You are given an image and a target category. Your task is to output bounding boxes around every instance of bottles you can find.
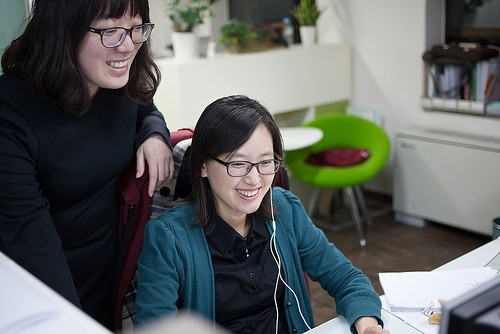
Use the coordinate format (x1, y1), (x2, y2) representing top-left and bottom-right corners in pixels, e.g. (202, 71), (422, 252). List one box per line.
(281, 17), (294, 50)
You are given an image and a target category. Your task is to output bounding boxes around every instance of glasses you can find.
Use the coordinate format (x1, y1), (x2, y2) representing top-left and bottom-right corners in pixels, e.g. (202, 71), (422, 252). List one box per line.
(88, 20), (156, 48)
(213, 152), (284, 177)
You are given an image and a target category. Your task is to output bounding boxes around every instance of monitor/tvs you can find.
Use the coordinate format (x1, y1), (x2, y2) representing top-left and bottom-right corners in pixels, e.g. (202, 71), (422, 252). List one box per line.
(435, 276), (500, 334)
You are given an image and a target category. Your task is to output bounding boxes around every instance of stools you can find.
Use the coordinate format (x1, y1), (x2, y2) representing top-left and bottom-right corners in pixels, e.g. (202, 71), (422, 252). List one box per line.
(284, 112), (390, 247)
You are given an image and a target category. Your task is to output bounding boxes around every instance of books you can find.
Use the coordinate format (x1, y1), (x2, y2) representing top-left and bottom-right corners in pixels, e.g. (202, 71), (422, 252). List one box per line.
(427, 58), (500, 103)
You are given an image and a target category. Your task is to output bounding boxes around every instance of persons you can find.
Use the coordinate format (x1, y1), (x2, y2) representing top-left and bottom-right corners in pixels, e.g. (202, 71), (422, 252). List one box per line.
(131, 95), (392, 334)
(0, 0), (176, 331)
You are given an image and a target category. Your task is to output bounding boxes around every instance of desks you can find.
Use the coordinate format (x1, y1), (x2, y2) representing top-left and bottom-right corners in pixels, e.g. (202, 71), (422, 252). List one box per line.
(303, 234), (500, 334)
(173, 124), (323, 155)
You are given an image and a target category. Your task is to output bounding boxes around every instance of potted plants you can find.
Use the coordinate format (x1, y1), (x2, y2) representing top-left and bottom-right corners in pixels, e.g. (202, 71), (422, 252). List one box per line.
(164, 0), (219, 60)
(290, 0), (323, 46)
(218, 17), (249, 54)
